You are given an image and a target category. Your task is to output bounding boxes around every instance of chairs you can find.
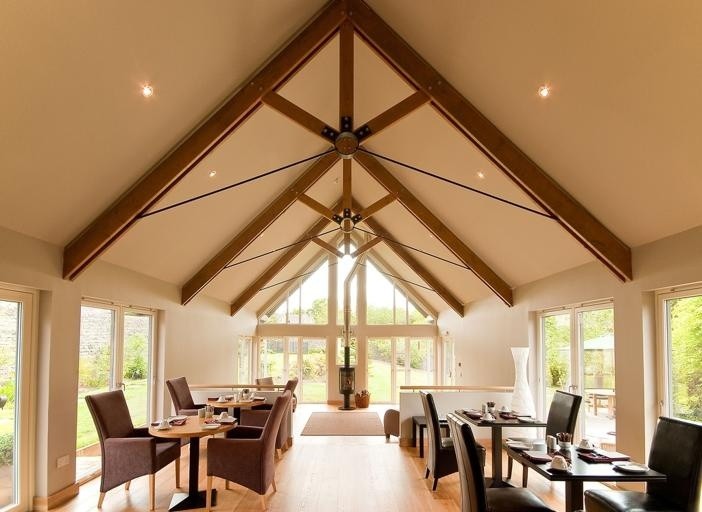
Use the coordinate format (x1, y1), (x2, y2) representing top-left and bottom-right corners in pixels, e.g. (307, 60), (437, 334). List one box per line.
(75, 366), (301, 512)
(584, 417), (702, 512)
(446, 412), (554, 512)
(421, 390), (485, 490)
(506, 390), (582, 488)
(384, 409), (399, 441)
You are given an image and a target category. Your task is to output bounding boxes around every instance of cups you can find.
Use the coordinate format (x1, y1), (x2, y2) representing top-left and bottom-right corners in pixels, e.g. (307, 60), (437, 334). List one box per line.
(217, 392), (265, 403)
(484, 413), (493, 418)
(546, 436), (556, 454)
(481, 403), (486, 412)
(156, 415), (169, 427)
(580, 438), (593, 447)
(552, 456), (566, 468)
(216, 407), (232, 418)
(196, 404), (214, 421)
(502, 405), (509, 410)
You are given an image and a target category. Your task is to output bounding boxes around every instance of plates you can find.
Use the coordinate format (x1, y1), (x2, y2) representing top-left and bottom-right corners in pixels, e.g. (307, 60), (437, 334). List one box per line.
(211, 418), (238, 424)
(168, 414), (189, 423)
(505, 434), (596, 471)
(461, 403), (542, 423)
(609, 460), (649, 473)
(154, 427), (173, 431)
(237, 400), (254, 402)
(254, 396), (264, 400)
(201, 423), (224, 429)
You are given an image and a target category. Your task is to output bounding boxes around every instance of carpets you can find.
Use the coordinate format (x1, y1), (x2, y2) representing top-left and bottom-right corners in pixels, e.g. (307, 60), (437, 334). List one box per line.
(301, 403), (388, 439)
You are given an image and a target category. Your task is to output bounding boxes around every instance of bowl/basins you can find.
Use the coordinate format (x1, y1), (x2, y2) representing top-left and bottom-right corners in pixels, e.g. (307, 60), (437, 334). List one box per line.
(488, 406), (495, 412)
(558, 441), (572, 450)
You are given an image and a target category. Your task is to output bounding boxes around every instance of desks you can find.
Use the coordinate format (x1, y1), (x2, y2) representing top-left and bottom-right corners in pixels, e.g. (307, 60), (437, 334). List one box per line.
(412, 416), (448, 459)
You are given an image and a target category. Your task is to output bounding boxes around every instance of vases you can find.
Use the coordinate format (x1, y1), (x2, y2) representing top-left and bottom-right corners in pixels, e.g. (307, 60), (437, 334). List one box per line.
(511, 345), (536, 444)
